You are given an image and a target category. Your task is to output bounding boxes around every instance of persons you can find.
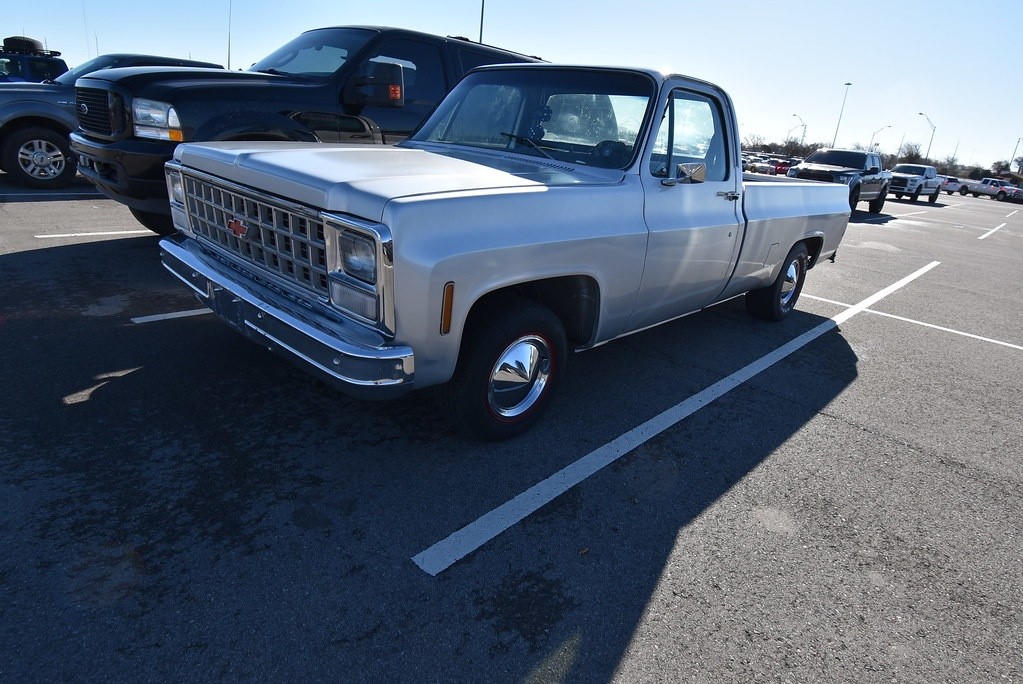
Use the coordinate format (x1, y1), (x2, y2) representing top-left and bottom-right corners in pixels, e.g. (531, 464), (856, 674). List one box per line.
(5, 62), (18, 76)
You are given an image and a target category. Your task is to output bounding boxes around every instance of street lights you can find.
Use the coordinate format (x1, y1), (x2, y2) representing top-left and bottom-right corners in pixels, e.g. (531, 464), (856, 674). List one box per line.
(1006, 137), (1023, 172)
(866, 124), (892, 153)
(918, 113), (937, 166)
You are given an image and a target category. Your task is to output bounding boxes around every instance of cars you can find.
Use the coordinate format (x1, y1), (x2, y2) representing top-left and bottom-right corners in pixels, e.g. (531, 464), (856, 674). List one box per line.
(788, 158), (802, 167)
(766, 158), (791, 177)
(742, 156), (776, 176)
(740, 151), (790, 164)
(618, 138), (713, 156)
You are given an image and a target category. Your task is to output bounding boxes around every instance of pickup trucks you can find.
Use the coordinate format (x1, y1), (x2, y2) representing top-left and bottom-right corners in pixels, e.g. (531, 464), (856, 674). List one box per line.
(157, 64), (852, 442)
(786, 149), (893, 215)
(960, 178), (1023, 203)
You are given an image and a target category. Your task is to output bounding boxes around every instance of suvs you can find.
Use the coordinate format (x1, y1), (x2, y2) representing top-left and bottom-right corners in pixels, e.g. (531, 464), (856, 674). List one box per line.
(70, 0), (620, 237)
(888, 164), (941, 204)
(935, 175), (961, 195)
(0, 54), (225, 191)
(0, 36), (70, 83)
